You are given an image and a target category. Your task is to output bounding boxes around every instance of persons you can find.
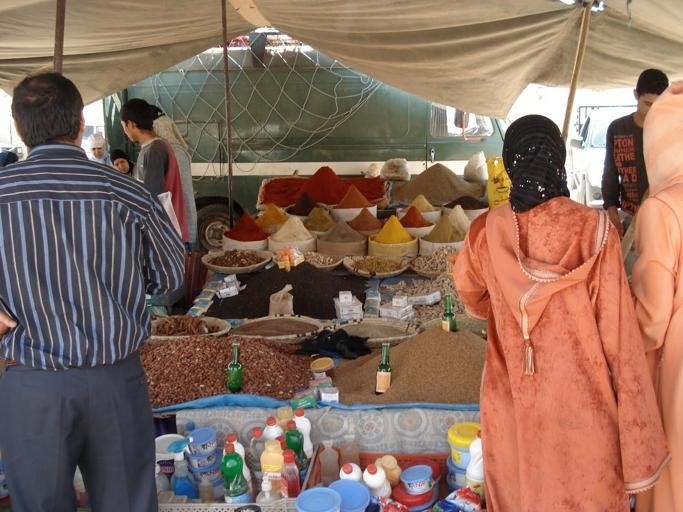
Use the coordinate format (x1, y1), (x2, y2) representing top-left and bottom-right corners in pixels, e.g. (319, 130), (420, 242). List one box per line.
(120, 96), (188, 245)
(602, 69), (683, 242)
(85, 132), (111, 165)
(150, 105), (197, 314)
(0, 71), (188, 510)
(453, 115), (671, 511)
(109, 148), (137, 177)
(626, 81), (681, 510)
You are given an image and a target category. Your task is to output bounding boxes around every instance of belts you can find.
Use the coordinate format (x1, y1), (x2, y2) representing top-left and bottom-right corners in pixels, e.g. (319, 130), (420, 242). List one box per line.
(7, 357), (18, 367)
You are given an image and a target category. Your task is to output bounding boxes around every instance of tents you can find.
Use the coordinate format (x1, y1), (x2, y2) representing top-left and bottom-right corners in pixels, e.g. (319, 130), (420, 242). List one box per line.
(0, 1), (683, 118)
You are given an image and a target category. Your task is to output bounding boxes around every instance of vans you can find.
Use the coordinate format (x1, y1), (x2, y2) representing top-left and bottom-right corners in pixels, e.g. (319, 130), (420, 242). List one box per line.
(79, 113), (105, 163)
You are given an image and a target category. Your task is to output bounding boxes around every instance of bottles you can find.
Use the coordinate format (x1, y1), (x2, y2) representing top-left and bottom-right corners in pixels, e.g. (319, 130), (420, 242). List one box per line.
(255, 477), (281, 503)
(375, 342), (391, 395)
(154, 464), (169, 495)
(262, 417), (283, 440)
(285, 421), (307, 464)
(275, 434), (287, 451)
(276, 406), (291, 430)
(259, 440), (283, 480)
(339, 463), (361, 483)
(375, 455), (401, 489)
(339, 434), (361, 464)
(291, 410), (313, 459)
(220, 444), (250, 503)
(227, 341), (242, 395)
(319, 440), (338, 486)
(222, 433), (253, 494)
(199, 473), (211, 501)
(249, 428), (264, 460)
(362, 464), (391, 500)
(169, 450), (199, 499)
(464, 430), (485, 503)
(281, 449), (300, 497)
(253, 466), (261, 495)
(442, 293), (455, 332)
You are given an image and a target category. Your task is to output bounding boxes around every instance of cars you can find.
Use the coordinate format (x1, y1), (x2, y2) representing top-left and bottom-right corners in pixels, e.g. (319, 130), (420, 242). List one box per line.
(565, 103), (636, 208)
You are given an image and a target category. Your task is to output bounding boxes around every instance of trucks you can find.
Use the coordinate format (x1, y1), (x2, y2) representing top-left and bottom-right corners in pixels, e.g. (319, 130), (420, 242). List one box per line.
(104, 30), (510, 252)
